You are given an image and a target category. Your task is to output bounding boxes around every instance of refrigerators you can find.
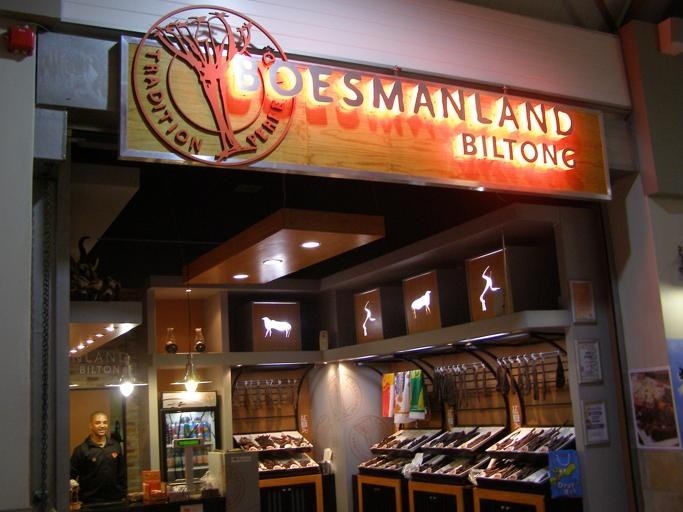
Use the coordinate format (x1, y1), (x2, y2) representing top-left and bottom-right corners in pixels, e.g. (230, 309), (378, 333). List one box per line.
(159, 390), (219, 481)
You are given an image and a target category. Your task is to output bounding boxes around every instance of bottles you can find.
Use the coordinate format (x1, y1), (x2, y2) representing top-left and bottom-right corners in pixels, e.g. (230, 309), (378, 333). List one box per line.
(166, 417), (212, 481)
(193, 327), (207, 352)
(165, 327), (177, 352)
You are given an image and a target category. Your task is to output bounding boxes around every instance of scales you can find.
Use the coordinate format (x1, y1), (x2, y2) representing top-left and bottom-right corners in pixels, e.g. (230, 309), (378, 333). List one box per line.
(166, 437), (208, 493)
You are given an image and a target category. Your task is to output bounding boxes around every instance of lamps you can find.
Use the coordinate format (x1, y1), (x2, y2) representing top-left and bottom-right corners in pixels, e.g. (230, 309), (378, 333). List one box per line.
(104, 335), (147, 398)
(170, 251), (210, 392)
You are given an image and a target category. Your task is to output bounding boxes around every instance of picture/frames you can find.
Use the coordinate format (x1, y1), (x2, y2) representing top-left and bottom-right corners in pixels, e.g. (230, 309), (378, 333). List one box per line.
(568, 279), (612, 450)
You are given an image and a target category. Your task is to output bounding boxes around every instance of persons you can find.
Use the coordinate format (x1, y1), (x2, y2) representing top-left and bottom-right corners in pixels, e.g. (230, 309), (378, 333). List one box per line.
(70, 411), (127, 504)
